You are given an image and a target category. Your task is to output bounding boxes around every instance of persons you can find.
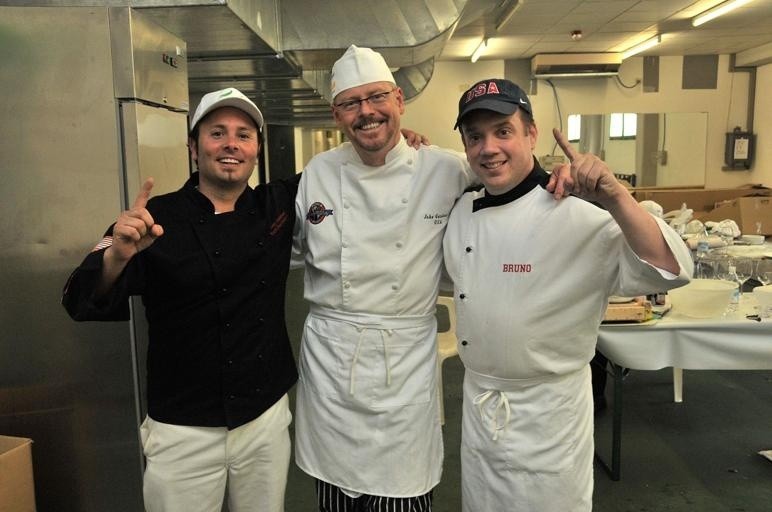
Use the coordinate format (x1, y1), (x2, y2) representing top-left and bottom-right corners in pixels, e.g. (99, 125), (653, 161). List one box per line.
(442, 77), (694, 511)
(289, 43), (589, 510)
(62, 86), (430, 512)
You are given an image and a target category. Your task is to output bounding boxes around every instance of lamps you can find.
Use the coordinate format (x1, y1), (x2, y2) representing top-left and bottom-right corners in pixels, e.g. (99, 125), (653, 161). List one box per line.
(691, 0), (754, 27)
(496, 0), (523, 31)
(622, 34), (661, 60)
(471, 39), (488, 64)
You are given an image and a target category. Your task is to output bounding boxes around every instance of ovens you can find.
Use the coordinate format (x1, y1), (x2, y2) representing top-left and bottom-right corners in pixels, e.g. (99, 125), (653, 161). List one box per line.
(0, 4), (187, 512)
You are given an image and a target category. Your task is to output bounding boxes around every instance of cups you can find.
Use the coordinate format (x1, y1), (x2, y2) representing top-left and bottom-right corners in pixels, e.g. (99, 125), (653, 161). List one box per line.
(753, 284), (772, 309)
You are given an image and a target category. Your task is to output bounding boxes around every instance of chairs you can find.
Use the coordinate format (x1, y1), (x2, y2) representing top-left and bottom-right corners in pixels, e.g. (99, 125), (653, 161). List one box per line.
(422, 295), (459, 427)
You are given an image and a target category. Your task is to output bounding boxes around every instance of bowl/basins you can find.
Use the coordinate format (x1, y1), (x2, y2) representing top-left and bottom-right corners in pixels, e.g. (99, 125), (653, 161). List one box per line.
(742, 234), (766, 245)
(671, 278), (740, 318)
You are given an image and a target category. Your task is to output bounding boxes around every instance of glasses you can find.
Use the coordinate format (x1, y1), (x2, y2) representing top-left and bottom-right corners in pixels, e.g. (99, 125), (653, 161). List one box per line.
(334, 90), (398, 109)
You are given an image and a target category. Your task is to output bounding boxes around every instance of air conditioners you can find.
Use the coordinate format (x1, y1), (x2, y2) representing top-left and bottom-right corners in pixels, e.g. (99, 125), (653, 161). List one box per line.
(530, 52), (622, 79)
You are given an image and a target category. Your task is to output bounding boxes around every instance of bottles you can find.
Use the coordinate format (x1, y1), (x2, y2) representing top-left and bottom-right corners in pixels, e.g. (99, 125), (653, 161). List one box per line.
(724, 266), (741, 316)
(697, 225), (708, 258)
(647, 292), (665, 305)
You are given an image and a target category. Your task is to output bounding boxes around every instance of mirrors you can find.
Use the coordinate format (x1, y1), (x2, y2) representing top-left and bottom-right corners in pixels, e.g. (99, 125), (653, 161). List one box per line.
(567, 112), (708, 188)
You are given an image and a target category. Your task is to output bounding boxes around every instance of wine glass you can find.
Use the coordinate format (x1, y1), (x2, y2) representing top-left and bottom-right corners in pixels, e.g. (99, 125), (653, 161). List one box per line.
(730, 258), (752, 306)
(756, 260), (772, 286)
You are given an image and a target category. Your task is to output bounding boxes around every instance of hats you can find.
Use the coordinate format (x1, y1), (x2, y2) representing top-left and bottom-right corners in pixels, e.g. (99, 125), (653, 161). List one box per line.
(454, 78), (534, 131)
(189, 87), (264, 135)
(330, 42), (397, 103)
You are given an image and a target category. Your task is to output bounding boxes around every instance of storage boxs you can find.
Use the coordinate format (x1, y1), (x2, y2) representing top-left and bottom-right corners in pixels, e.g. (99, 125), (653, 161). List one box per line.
(0, 435), (37, 512)
(715, 192), (772, 236)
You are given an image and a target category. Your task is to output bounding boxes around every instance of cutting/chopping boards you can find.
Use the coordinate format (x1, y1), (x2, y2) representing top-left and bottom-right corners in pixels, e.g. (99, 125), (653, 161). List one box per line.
(604, 293), (654, 325)
